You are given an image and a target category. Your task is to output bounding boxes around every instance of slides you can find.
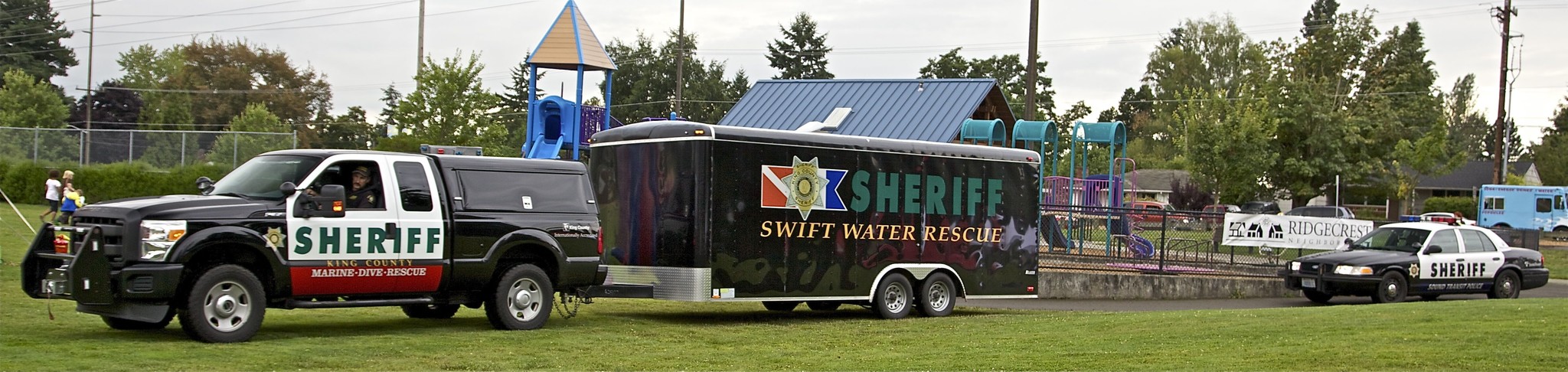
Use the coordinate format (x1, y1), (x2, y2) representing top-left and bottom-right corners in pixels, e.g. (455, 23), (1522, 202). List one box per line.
(527, 135), (564, 159)
(1040, 214), (1076, 249)
(1105, 215), (1155, 257)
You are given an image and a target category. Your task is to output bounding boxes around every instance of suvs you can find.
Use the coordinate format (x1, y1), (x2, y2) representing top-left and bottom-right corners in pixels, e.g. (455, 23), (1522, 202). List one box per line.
(1124, 201), (1195, 231)
(1201, 202), (1355, 231)
(20, 144), (608, 344)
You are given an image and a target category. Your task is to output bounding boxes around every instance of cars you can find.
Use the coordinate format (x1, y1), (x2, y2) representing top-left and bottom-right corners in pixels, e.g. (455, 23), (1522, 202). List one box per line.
(1277, 215), (1549, 303)
(1420, 213), (1476, 226)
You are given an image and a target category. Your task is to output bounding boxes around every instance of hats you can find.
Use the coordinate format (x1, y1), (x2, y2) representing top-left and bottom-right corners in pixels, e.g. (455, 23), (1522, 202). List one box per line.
(352, 167), (371, 177)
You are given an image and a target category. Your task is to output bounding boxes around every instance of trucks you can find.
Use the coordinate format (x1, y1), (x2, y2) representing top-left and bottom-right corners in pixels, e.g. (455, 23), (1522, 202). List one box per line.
(1476, 184), (1568, 241)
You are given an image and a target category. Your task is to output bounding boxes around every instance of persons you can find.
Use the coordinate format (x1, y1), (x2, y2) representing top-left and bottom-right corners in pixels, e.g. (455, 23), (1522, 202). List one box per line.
(39, 169), (88, 227)
(302, 166), (376, 210)
